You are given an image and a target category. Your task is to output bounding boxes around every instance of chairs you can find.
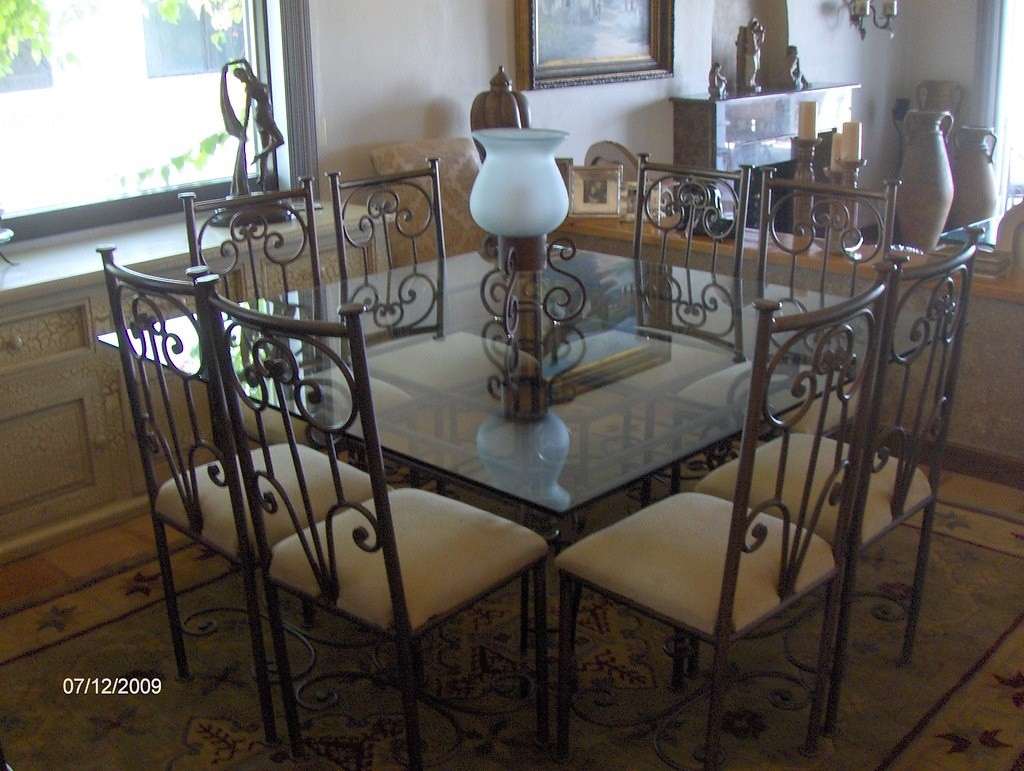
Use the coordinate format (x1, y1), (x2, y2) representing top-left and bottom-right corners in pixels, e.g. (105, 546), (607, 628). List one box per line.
(94, 152), (984, 771)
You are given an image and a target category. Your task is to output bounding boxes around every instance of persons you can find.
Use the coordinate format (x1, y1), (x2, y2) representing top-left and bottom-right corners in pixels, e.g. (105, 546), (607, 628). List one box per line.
(744, 17), (766, 86)
(228, 60), (285, 194)
(587, 180), (606, 204)
(778, 46), (812, 91)
(709, 63), (728, 100)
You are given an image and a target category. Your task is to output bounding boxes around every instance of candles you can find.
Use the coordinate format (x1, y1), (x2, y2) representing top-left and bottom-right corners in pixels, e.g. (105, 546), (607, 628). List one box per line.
(841, 122), (862, 160)
(830, 132), (850, 174)
(797, 100), (817, 140)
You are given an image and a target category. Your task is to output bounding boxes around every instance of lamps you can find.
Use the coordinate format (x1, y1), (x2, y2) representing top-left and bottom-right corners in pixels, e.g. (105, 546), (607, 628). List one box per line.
(477, 317), (585, 512)
(850, 0), (899, 40)
(470, 122), (586, 338)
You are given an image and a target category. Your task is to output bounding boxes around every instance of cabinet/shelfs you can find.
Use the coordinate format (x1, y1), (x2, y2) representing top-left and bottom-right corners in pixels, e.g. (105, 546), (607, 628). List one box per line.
(0, 198), (408, 569)
(669, 82), (863, 237)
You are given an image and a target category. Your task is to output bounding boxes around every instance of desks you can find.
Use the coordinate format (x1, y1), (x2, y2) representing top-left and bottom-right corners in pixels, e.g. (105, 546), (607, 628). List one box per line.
(95, 242), (973, 546)
(547, 211), (948, 444)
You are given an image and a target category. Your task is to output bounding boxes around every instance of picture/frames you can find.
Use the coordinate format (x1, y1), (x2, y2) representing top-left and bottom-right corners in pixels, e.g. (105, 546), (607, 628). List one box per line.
(555, 158), (572, 191)
(568, 165), (623, 218)
(618, 179), (661, 223)
(512, 0), (675, 92)
